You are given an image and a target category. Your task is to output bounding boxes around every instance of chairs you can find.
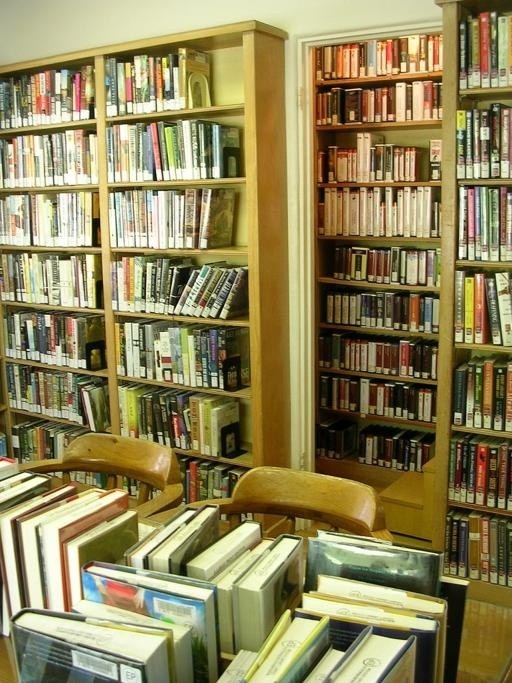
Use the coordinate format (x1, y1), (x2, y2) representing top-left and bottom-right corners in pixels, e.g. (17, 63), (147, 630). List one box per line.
(147, 464), (392, 553)
(16, 434), (184, 515)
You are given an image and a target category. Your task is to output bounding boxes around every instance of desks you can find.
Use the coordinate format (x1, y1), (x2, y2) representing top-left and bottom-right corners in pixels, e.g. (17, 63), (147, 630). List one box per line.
(1, 457), (512, 683)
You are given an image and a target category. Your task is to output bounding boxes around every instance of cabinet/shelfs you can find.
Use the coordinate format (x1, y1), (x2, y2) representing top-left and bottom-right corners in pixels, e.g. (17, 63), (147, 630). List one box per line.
(439, 1), (512, 587)
(102, 21), (294, 538)
(304, 24), (444, 489)
(1, 47), (113, 481)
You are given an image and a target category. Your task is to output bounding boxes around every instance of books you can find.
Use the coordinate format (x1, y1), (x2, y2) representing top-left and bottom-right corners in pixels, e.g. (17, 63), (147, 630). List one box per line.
(1, 456), (469, 683)
(316, 32), (444, 472)
(445, 13), (512, 587)
(104, 46), (247, 503)
(0, 66), (110, 488)
